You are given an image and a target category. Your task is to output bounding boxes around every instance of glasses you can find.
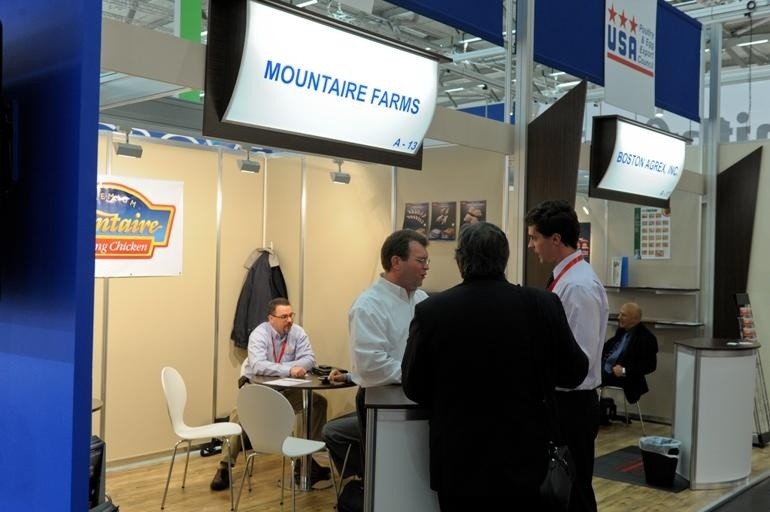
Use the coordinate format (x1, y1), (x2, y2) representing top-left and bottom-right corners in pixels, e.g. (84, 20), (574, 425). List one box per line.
(271, 312), (296, 320)
(404, 253), (432, 265)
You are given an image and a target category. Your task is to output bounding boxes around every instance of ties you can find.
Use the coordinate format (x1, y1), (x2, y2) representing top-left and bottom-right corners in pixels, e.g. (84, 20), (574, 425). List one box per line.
(605, 331), (628, 374)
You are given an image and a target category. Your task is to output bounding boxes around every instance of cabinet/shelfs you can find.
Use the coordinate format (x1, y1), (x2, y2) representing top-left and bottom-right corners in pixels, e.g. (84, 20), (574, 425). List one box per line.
(600, 285), (704, 327)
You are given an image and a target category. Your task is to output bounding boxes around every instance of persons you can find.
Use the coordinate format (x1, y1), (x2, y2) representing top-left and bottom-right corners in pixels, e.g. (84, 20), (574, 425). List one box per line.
(599, 300), (659, 386)
(209, 298), (331, 491)
(320, 367), (367, 511)
(517, 199), (611, 511)
(345, 228), (430, 441)
(400, 220), (591, 512)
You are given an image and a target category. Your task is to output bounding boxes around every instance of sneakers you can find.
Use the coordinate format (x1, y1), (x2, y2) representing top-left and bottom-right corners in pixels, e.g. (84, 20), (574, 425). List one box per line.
(209, 469), (231, 491)
(293, 457), (331, 480)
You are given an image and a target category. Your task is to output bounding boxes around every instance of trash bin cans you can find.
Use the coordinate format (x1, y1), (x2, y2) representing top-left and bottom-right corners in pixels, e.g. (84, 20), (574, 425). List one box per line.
(640, 436), (682, 487)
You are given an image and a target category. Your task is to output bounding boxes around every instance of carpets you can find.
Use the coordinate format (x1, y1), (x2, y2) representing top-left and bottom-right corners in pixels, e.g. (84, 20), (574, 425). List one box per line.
(593, 445), (690, 493)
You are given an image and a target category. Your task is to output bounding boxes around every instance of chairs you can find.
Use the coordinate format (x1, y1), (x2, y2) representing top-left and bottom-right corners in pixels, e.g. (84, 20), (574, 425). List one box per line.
(235, 384), (337, 511)
(598, 387), (647, 436)
(161, 366), (252, 510)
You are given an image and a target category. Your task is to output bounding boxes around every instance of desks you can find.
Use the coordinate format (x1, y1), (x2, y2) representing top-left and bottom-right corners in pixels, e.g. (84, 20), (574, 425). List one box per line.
(250, 375), (357, 492)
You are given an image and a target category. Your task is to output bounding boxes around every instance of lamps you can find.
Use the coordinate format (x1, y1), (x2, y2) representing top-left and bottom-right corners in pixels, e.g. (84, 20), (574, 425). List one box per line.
(237, 144), (260, 173)
(329, 158), (350, 184)
(112, 124), (143, 159)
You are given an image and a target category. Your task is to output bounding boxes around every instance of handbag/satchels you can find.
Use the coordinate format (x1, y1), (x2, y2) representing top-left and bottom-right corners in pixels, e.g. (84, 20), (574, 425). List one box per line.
(541, 431), (581, 509)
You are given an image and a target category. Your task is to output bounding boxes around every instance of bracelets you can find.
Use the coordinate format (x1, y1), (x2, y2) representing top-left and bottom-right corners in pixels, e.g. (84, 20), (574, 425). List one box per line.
(621, 367), (626, 376)
